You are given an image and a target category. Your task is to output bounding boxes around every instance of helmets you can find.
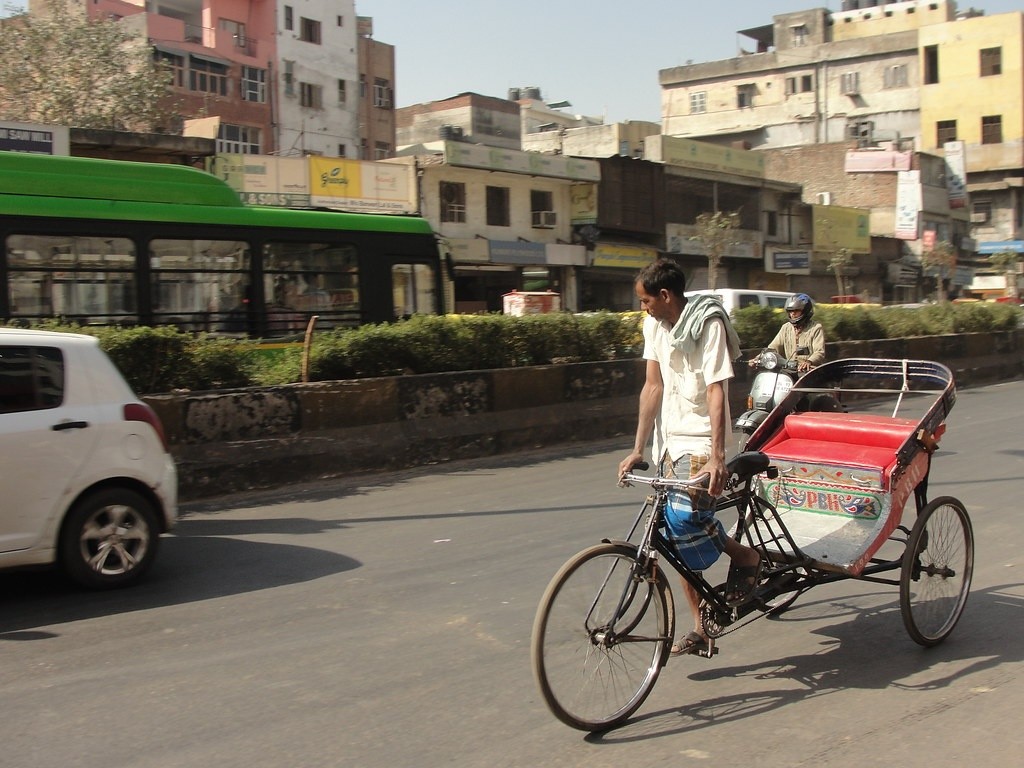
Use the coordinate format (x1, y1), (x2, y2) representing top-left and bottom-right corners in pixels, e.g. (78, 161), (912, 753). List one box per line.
(784, 293), (814, 327)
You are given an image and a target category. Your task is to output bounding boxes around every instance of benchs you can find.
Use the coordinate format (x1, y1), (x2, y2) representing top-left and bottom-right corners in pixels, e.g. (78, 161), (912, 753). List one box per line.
(756, 411), (931, 514)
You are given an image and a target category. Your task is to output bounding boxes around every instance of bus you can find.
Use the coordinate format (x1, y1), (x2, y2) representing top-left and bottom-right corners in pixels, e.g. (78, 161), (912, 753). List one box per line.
(0, 149), (460, 346)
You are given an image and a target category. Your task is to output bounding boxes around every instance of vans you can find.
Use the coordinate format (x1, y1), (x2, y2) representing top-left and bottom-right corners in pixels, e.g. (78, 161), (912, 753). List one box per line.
(684, 288), (816, 326)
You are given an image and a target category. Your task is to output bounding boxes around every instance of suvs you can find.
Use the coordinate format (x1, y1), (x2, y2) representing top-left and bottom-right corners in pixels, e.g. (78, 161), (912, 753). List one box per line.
(0, 325), (180, 594)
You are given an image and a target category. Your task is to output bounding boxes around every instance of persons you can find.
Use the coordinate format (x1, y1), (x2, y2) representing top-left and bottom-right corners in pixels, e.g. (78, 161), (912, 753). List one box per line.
(618, 259), (764, 658)
(749, 293), (825, 400)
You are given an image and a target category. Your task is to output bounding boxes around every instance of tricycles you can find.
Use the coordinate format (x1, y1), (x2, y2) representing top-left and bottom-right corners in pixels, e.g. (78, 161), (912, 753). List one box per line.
(530, 356), (976, 734)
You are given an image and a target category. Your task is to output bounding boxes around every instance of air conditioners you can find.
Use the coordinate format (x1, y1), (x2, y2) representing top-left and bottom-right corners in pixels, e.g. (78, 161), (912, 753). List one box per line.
(815, 191), (831, 206)
(531, 211), (556, 228)
(970, 213), (987, 223)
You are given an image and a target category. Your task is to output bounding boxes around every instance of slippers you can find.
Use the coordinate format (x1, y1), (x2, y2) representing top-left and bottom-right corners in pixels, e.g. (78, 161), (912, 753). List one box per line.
(725, 554), (763, 606)
(669, 631), (718, 657)
